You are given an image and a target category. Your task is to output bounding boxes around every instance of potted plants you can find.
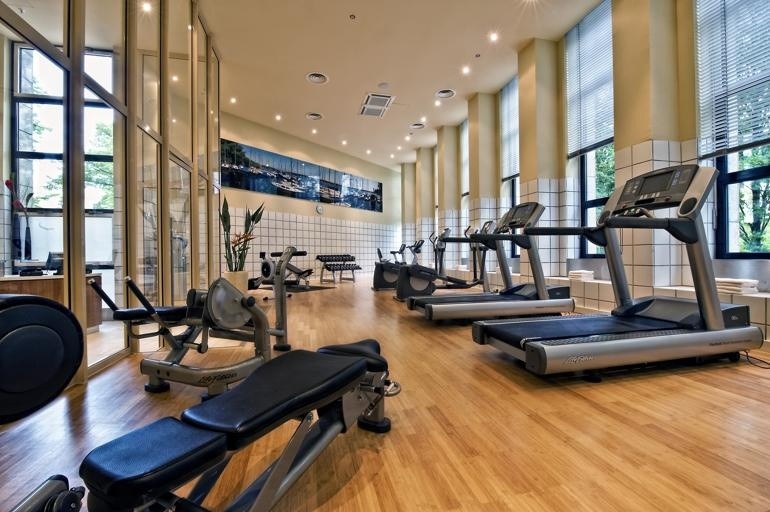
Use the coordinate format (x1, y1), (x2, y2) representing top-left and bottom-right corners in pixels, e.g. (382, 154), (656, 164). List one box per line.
(217, 192), (267, 298)
(136, 194), (191, 302)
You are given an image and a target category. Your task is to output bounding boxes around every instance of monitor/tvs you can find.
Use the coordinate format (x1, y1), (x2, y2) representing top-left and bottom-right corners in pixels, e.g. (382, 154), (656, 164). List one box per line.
(634, 168), (677, 196)
(44, 252), (63, 275)
(511, 204), (529, 219)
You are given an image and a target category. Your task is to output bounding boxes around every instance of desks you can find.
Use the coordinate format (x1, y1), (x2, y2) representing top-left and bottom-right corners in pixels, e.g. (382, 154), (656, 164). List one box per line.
(0, 272), (103, 335)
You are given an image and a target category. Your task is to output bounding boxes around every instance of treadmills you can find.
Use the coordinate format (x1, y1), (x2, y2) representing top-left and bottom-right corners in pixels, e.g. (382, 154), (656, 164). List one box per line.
(408, 202), (576, 320)
(472, 164), (764, 374)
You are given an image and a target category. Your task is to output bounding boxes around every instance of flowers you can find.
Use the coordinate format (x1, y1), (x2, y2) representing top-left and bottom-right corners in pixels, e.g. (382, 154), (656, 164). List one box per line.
(4, 179), (35, 228)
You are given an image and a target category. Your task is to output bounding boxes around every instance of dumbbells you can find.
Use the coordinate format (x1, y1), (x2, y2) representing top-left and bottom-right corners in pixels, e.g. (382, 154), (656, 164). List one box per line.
(324, 264), (362, 271)
(317, 255), (355, 262)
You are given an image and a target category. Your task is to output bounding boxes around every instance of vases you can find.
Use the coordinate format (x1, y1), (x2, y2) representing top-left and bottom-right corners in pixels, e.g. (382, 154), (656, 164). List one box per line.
(24, 227), (32, 261)
